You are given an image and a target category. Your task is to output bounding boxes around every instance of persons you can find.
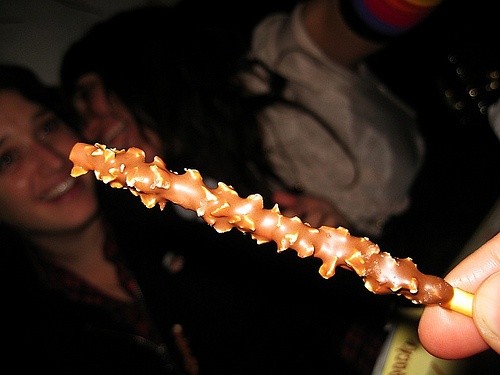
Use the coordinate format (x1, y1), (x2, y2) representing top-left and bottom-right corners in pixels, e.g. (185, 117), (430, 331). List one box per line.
(417, 228), (499, 362)
(62, 2), (499, 320)
(1, 61), (263, 374)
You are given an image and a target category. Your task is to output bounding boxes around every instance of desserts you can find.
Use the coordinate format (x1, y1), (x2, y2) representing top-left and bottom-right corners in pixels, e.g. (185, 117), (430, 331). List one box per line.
(69, 142), (477, 319)
